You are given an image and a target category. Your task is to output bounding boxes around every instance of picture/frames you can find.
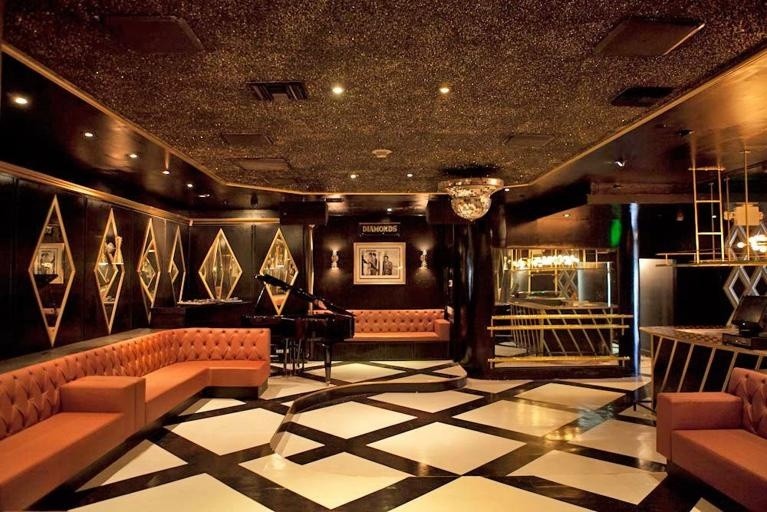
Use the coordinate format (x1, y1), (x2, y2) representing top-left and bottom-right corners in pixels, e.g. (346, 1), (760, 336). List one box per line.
(353, 242), (406, 285)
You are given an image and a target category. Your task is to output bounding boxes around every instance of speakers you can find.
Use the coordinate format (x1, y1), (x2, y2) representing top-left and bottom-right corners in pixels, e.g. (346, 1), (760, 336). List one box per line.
(425, 200), (475, 224)
(280, 201), (328, 225)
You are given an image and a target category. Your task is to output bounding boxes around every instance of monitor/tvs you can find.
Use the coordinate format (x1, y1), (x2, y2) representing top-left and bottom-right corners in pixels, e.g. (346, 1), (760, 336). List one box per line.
(731, 295), (767, 335)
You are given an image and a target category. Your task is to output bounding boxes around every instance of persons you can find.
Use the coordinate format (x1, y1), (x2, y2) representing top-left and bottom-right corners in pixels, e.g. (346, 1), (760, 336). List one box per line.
(381, 255), (393, 275)
(369, 253), (377, 275)
(366, 252), (373, 263)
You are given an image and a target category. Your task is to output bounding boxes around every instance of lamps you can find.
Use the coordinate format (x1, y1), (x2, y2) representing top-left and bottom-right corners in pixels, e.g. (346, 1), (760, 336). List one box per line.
(511, 252), (579, 272)
(436, 173), (503, 223)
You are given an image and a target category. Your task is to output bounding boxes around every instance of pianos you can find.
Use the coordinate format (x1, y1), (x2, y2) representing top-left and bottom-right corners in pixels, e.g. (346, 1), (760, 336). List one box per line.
(240, 272), (357, 386)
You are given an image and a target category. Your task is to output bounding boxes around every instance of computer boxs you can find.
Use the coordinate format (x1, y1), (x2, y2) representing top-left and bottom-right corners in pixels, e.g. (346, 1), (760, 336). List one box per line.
(722, 332), (767, 347)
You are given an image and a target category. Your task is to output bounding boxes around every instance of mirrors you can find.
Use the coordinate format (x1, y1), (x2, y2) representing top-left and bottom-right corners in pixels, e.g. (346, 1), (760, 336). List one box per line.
(495, 245), (619, 361)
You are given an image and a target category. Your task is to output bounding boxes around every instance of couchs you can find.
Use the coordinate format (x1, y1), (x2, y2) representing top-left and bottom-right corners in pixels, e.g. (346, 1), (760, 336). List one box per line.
(656, 367), (765, 511)
(307, 308), (451, 361)
(0, 327), (271, 510)
